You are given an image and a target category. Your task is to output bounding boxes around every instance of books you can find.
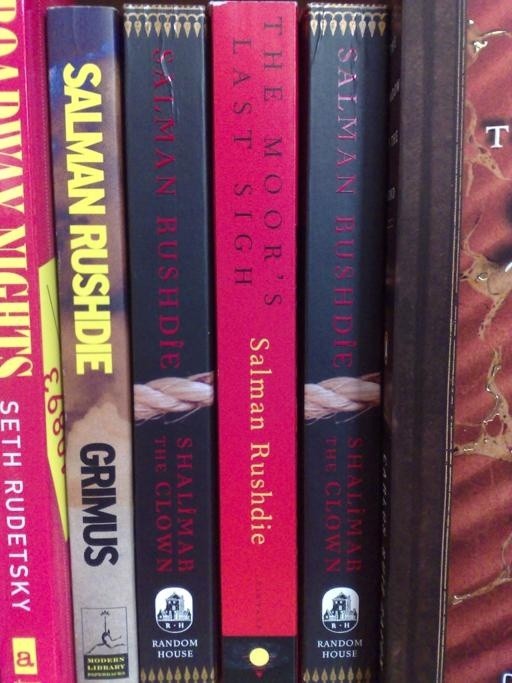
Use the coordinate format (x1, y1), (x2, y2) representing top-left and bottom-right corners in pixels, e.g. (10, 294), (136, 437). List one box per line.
(1, 1), (512, 683)
